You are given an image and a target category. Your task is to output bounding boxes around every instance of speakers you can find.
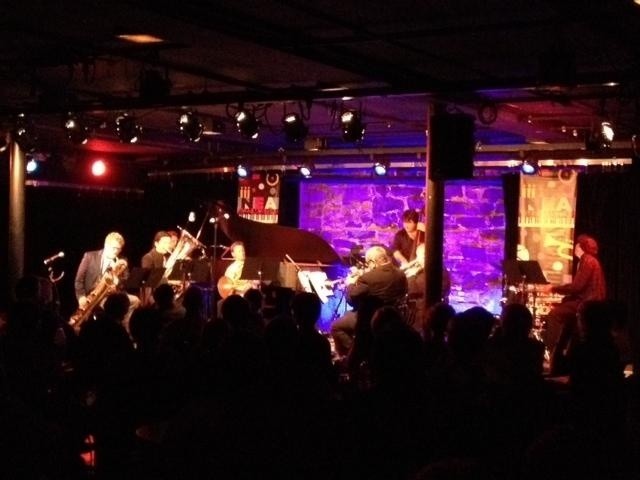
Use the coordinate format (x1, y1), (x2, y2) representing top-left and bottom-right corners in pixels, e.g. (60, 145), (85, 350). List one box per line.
(428, 112), (474, 181)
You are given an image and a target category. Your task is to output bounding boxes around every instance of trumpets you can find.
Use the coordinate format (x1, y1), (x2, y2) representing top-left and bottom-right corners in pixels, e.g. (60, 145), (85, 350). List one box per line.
(324, 270), (363, 290)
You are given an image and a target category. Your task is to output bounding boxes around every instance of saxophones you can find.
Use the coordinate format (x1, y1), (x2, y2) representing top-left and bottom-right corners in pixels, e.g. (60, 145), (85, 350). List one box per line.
(69, 258), (127, 327)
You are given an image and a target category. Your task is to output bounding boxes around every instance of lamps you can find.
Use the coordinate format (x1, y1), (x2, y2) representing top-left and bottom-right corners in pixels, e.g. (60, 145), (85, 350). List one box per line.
(1, 83), (370, 153)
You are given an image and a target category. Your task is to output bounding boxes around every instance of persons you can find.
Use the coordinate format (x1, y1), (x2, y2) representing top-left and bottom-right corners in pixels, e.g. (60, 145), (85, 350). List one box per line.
(542, 236), (607, 363)
(1, 272), (639, 479)
(164, 232), (189, 287)
(391, 210), (430, 323)
(75, 232), (129, 308)
(142, 230), (183, 297)
(221, 241), (261, 301)
(331, 244), (408, 354)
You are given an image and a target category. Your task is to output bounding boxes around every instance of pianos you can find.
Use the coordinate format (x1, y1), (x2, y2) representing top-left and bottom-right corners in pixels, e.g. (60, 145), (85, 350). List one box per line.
(215, 201), (348, 296)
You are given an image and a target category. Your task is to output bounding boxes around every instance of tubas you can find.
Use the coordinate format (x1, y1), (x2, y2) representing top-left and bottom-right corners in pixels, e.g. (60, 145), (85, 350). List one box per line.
(158, 226), (206, 304)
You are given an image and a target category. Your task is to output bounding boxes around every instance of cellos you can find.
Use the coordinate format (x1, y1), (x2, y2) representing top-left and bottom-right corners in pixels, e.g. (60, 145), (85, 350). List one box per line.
(400, 207), (450, 336)
